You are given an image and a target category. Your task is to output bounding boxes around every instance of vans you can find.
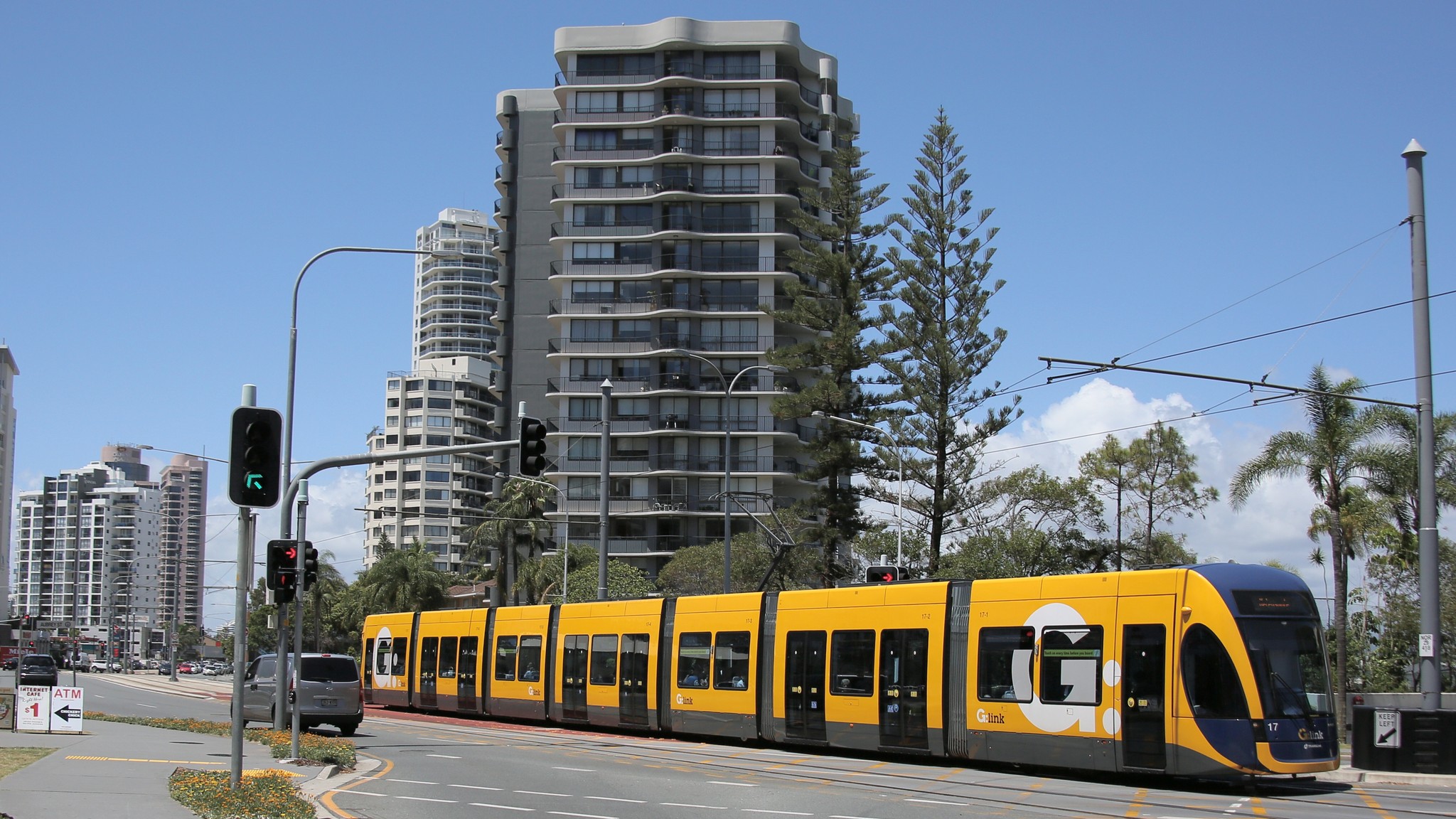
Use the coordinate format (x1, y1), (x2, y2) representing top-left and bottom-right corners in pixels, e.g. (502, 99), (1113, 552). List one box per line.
(69, 651), (90, 673)
(229, 652), (365, 737)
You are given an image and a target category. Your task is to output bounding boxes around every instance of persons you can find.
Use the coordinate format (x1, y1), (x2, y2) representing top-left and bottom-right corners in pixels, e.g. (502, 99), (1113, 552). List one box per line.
(682, 666), (708, 687)
(523, 662), (538, 679)
(840, 679), (851, 692)
(737, 670), (747, 687)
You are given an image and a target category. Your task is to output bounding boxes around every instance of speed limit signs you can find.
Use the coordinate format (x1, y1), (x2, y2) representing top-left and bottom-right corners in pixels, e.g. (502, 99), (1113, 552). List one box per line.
(171, 632), (179, 642)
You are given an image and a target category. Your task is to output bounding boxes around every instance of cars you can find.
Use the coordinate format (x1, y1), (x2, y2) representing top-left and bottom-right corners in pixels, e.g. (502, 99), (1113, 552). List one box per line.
(127, 655), (255, 677)
(2, 658), (18, 671)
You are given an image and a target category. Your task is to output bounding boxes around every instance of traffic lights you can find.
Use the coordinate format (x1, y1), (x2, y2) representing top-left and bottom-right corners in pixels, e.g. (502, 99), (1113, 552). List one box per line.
(518, 416), (547, 477)
(303, 540), (319, 592)
(74, 639), (77, 647)
(225, 406), (285, 509)
(865, 565), (899, 583)
(272, 545), (299, 604)
(101, 643), (104, 650)
(897, 566), (910, 581)
(29, 641), (34, 647)
(24, 614), (30, 625)
(75, 648), (78, 656)
(101, 651), (104, 657)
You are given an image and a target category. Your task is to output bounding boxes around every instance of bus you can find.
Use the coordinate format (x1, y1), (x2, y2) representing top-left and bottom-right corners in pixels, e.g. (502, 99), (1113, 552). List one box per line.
(358, 561), (1340, 788)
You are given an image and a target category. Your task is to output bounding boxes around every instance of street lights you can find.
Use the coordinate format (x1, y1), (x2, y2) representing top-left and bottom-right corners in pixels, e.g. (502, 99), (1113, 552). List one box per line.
(178, 610), (231, 666)
(72, 548), (182, 675)
(666, 347), (790, 596)
(205, 616), (236, 640)
(275, 247), (463, 737)
(141, 603), (203, 665)
(74, 584), (158, 674)
(495, 471), (568, 605)
(64, 569), (158, 674)
(812, 410), (902, 568)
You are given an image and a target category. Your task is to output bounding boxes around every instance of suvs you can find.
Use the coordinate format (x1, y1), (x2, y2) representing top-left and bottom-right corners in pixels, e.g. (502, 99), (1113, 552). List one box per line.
(15, 653), (58, 690)
(91, 660), (122, 674)
(96, 504), (260, 682)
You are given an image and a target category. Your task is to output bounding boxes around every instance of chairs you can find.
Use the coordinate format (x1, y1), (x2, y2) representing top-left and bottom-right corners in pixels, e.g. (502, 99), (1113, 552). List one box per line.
(418, 668), (1019, 699)
